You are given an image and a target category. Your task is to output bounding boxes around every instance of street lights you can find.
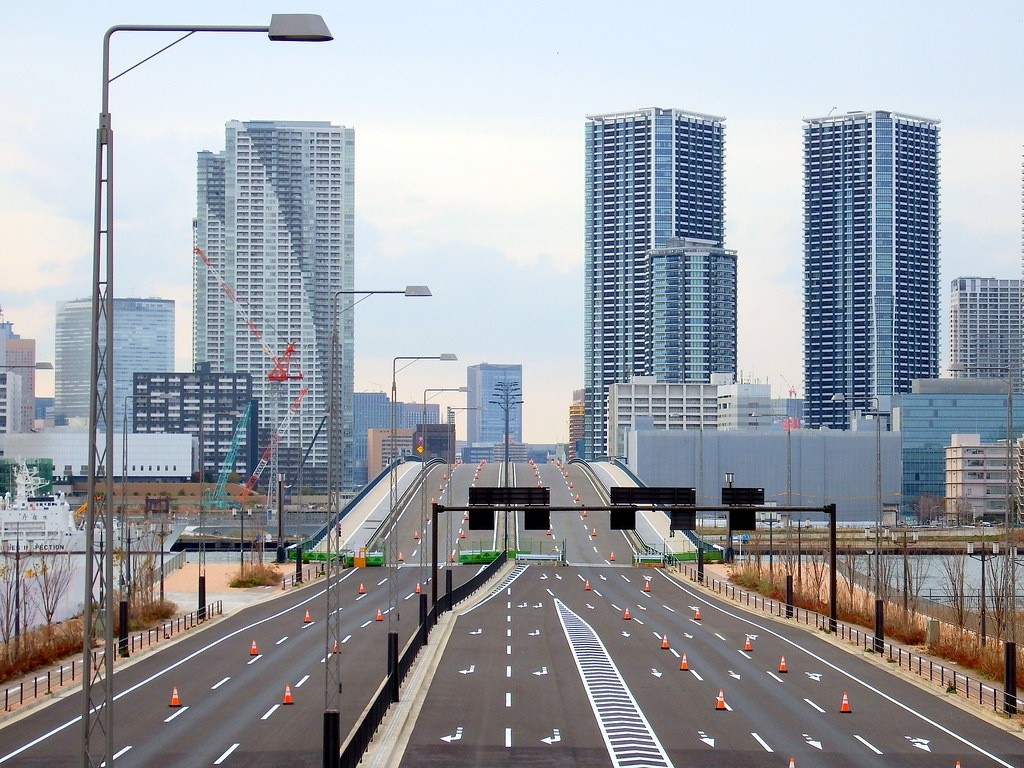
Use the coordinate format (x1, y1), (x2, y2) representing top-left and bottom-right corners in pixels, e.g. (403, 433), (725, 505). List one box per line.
(751, 412), (794, 619)
(382, 354), (460, 702)
(323, 283), (435, 768)
(445, 405), (488, 614)
(79, 11), (334, 768)
(418, 386), (471, 645)
(117, 394), (172, 659)
(830, 395), (886, 658)
(668, 412), (704, 583)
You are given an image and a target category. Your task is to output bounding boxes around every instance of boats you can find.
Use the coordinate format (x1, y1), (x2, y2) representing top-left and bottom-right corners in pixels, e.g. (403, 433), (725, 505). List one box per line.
(0, 455), (191, 553)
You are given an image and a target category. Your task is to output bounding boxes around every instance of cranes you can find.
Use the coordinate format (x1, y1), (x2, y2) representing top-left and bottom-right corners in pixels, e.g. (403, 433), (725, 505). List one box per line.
(193, 246), (310, 509)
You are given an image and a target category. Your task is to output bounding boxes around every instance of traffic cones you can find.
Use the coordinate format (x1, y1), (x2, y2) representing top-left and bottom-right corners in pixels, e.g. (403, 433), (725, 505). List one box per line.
(839, 690), (852, 713)
(779, 655), (788, 672)
(789, 758), (795, 768)
(167, 687), (182, 707)
(416, 580), (420, 593)
(660, 633), (670, 649)
(716, 689), (726, 710)
(956, 761), (961, 768)
(679, 652), (690, 671)
(584, 579), (592, 591)
(333, 640), (342, 653)
(250, 639), (259, 656)
(694, 608), (702, 620)
(644, 581), (651, 591)
(304, 608), (311, 624)
(358, 581), (366, 595)
(623, 607), (632, 620)
(282, 683), (294, 705)
(396, 459), (487, 563)
(529, 459), (617, 562)
(744, 635), (753, 651)
(376, 607), (385, 621)
